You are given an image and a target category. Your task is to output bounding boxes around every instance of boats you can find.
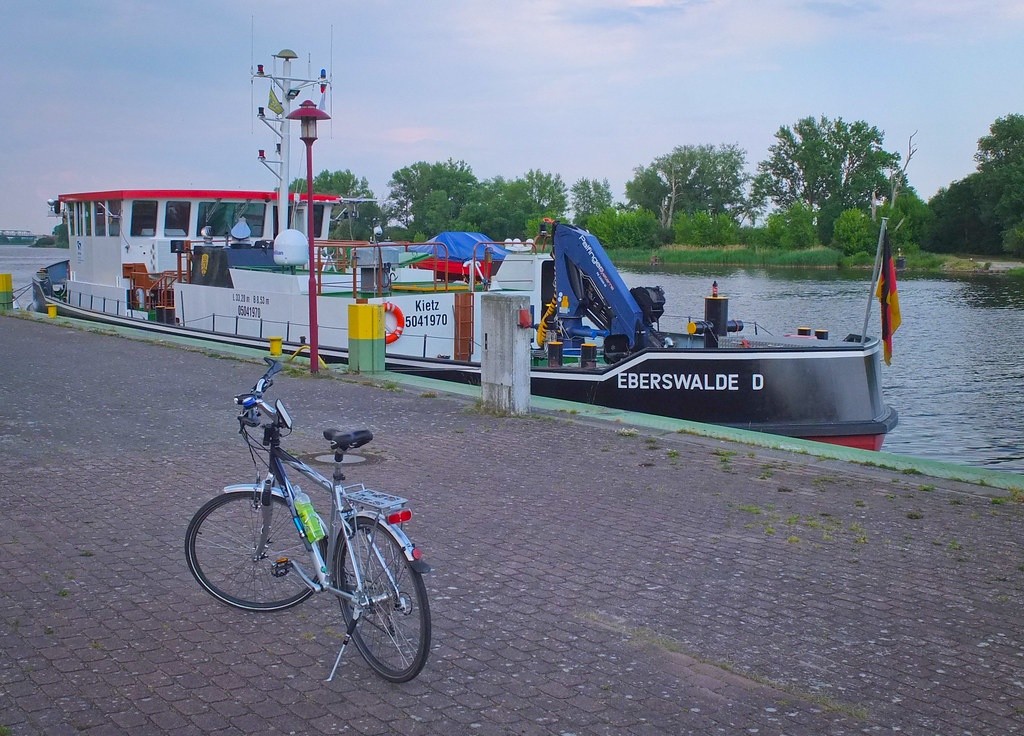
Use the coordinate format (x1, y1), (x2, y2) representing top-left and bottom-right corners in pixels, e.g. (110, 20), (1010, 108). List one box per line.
(406, 230), (511, 282)
(29, 10), (900, 453)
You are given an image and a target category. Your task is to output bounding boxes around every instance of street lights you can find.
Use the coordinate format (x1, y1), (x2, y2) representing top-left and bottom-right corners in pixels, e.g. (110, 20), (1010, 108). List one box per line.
(284, 97), (331, 374)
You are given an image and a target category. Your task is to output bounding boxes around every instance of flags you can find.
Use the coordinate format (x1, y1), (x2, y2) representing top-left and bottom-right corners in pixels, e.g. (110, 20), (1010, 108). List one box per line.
(874, 232), (901, 368)
(268, 90), (284, 115)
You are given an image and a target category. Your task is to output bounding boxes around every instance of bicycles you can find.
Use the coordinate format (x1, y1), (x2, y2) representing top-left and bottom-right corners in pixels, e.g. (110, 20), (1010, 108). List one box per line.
(182, 355), (434, 685)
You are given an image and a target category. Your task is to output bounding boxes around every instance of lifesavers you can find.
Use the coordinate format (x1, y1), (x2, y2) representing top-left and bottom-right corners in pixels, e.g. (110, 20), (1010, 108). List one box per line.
(383, 302), (405, 344)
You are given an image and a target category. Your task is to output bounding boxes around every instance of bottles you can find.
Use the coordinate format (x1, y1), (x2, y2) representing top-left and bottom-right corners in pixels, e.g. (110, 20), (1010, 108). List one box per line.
(293, 484), (327, 544)
